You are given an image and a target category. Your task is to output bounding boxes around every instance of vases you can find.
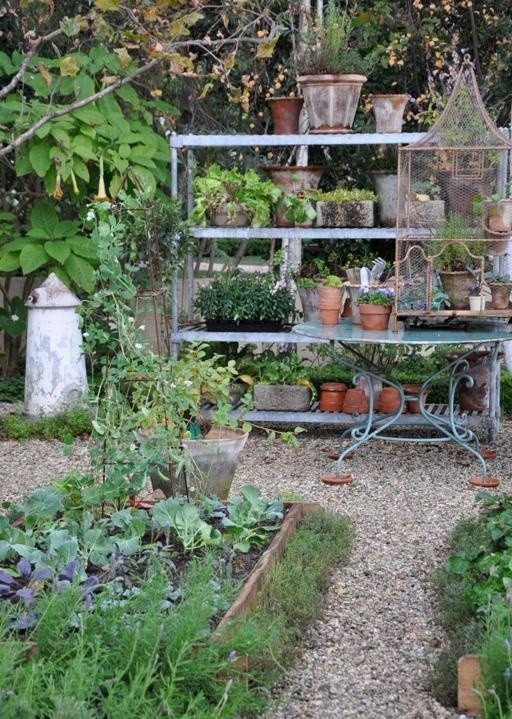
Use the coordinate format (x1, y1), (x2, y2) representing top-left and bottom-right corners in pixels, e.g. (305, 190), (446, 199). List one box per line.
(264, 160), (323, 224)
(370, 165), (427, 222)
(369, 95), (407, 131)
(344, 282), (394, 323)
(445, 351), (504, 414)
(404, 384), (430, 412)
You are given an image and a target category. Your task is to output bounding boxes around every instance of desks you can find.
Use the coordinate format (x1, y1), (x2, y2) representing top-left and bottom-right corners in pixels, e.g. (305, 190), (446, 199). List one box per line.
(294, 319), (511, 487)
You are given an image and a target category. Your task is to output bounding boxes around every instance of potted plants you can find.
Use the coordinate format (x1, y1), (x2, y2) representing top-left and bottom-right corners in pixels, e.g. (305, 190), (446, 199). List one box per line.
(464, 280), (488, 311)
(437, 85), (493, 225)
(399, 281), (447, 307)
(355, 340), (399, 410)
(183, 165), (275, 228)
(140, 349), (252, 498)
(290, 257), (348, 326)
(302, 8), (369, 135)
(246, 347), (317, 410)
(264, 69), (304, 133)
(317, 272), (344, 324)
(357, 286), (393, 332)
(431, 241), (485, 309)
(401, 180), (445, 228)
(484, 271), (511, 309)
(316, 187), (376, 228)
(191, 266), (294, 334)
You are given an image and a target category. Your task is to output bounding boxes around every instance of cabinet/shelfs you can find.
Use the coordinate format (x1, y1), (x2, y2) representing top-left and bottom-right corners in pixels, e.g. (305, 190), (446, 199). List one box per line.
(162, 123), (511, 439)
